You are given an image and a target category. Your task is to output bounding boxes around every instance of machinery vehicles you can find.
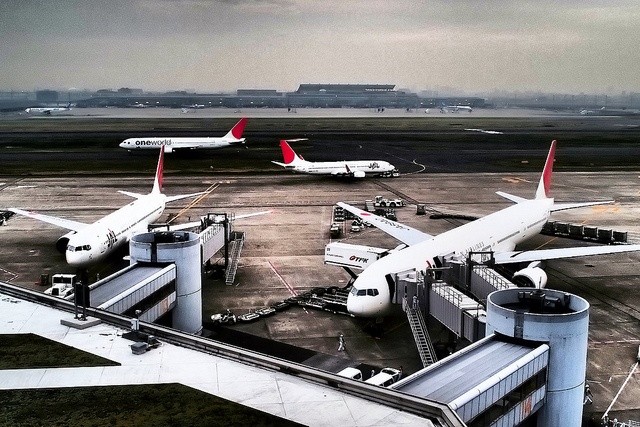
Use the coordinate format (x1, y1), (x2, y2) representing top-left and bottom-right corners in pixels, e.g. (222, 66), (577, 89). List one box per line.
(210, 312), (237, 325)
(44, 273), (82, 300)
(351, 221), (360, 231)
(380, 168), (400, 177)
(374, 195), (406, 207)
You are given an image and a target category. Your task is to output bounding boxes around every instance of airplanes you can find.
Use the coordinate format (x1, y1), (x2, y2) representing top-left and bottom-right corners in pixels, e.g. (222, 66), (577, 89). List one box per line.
(1, 143), (274, 266)
(580, 106), (607, 114)
(271, 136), (396, 177)
(336, 140), (640, 317)
(25, 101), (75, 116)
(118, 117), (248, 153)
(440, 104), (473, 113)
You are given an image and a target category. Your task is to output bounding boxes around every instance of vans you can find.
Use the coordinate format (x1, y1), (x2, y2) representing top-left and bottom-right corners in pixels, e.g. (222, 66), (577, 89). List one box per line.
(365, 367), (401, 387)
(336, 366), (363, 380)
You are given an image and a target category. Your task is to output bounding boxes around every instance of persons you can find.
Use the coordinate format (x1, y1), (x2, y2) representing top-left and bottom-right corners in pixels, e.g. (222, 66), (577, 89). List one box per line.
(583, 384), (593, 406)
(337, 333), (347, 353)
(602, 412), (610, 424)
(369, 369), (375, 377)
(398, 365), (403, 374)
(3, 216), (8, 226)
